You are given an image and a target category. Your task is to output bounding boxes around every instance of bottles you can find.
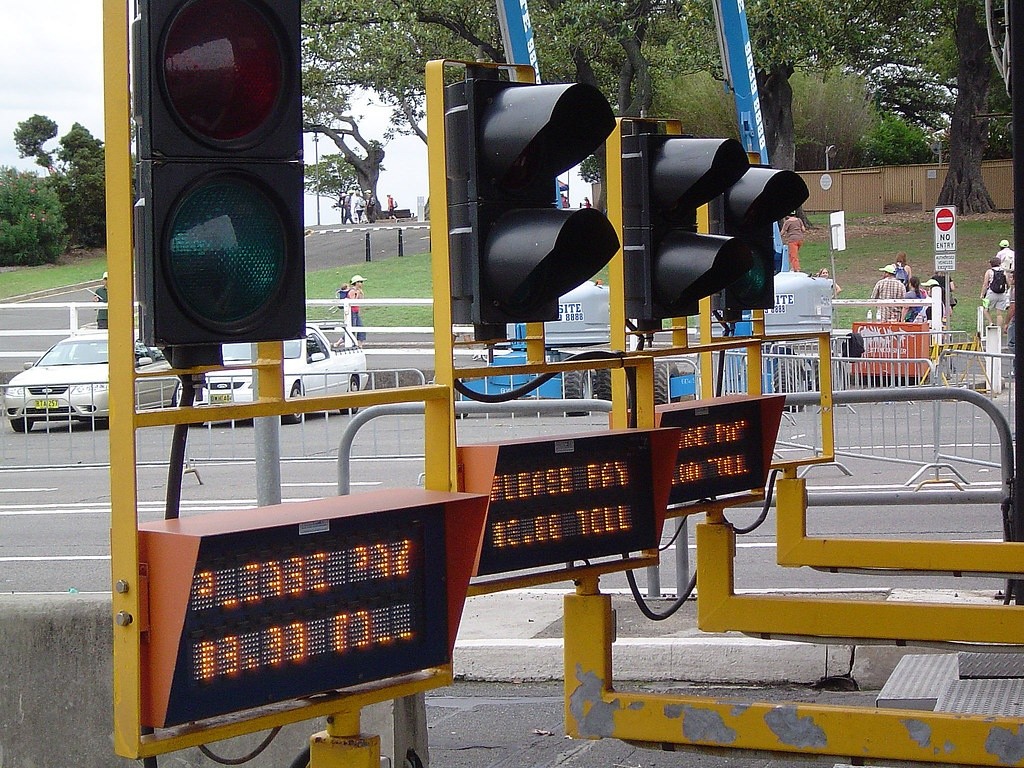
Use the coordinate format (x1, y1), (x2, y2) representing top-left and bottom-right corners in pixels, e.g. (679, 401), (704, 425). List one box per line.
(876, 311), (881, 322)
(867, 310), (872, 323)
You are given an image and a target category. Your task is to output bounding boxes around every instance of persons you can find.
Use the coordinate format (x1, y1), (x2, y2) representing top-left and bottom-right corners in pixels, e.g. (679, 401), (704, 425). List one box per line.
(981, 257), (1006, 326)
(93, 272), (108, 329)
(818, 268), (841, 311)
(343, 190), (376, 224)
(1004, 271), (1014, 376)
(584, 197), (590, 208)
(871, 251), (955, 343)
(997, 240), (1015, 310)
(341, 275), (368, 347)
(561, 194), (567, 208)
(387, 194), (396, 219)
(780, 209), (806, 272)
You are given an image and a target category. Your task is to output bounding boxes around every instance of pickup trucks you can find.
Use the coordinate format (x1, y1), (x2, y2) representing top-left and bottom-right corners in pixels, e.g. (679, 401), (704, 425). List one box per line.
(173, 319), (369, 429)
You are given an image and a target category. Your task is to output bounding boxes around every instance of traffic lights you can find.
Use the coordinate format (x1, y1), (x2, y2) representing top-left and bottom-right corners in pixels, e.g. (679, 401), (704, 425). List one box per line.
(620, 127), (751, 319)
(709, 162), (809, 312)
(441, 79), (623, 325)
(132, 0), (308, 349)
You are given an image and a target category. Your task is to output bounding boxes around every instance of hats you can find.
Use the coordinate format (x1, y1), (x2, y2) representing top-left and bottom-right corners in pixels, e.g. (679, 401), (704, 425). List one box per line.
(879, 265), (895, 274)
(982, 298), (990, 308)
(999, 240), (1009, 248)
(921, 279), (940, 287)
(103, 272), (108, 279)
(351, 275), (367, 284)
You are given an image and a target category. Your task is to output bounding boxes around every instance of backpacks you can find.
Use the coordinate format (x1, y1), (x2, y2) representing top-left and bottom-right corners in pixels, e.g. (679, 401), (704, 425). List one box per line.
(895, 263), (908, 287)
(989, 268), (1005, 293)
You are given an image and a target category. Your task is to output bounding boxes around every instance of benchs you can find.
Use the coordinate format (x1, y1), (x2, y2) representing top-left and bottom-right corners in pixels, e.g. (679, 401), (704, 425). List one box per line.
(382, 209), (415, 219)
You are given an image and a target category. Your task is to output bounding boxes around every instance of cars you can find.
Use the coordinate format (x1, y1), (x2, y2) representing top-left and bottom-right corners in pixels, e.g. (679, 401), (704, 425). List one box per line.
(4, 329), (184, 432)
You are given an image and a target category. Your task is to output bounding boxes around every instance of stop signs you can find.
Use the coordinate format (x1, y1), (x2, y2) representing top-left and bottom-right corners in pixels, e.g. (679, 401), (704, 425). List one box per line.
(934, 205), (958, 252)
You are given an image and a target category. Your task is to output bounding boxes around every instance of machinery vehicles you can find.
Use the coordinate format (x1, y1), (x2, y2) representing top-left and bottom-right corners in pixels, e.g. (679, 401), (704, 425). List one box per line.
(454, 1), (837, 419)
